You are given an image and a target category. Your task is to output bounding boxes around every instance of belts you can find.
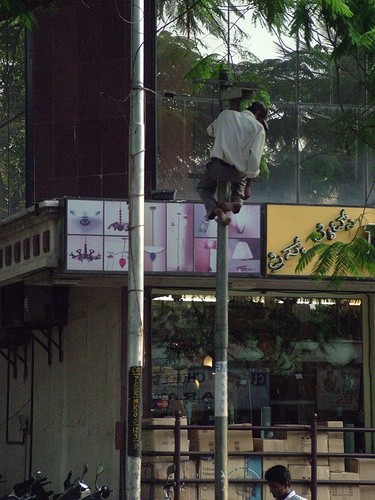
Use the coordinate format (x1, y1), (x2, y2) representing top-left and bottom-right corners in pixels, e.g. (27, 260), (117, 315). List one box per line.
(211, 157), (235, 170)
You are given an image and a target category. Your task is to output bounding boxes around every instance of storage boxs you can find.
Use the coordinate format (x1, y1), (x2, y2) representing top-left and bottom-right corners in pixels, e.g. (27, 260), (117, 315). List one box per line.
(141, 400), (375, 500)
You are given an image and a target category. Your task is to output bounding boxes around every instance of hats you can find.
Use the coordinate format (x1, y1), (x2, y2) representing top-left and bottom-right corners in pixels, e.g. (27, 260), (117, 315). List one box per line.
(252, 100), (272, 130)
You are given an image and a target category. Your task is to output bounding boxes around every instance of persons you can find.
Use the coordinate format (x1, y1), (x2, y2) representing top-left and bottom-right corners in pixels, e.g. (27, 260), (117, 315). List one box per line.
(150, 335), (364, 421)
(264, 463), (307, 500)
(194, 97), (270, 226)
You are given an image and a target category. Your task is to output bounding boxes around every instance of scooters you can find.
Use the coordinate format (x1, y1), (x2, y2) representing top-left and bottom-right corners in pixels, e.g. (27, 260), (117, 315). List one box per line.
(0, 463), (113, 500)
